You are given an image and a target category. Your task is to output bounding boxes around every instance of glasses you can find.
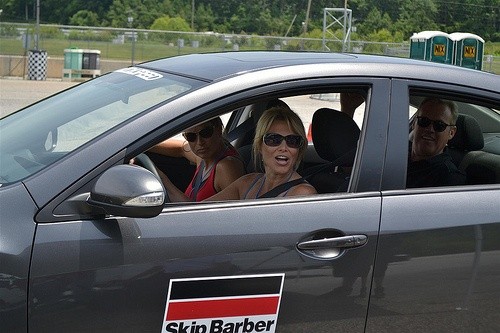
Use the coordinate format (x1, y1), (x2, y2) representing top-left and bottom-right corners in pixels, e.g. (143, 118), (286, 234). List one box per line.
(182, 124), (218, 143)
(415, 115), (456, 132)
(262, 133), (305, 148)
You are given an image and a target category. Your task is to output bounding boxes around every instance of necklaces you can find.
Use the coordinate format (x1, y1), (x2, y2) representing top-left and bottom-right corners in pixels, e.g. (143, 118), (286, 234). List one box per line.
(129, 107), (318, 202)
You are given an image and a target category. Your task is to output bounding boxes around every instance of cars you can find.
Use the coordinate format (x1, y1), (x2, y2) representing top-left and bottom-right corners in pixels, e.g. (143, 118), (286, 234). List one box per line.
(0, 49), (500, 333)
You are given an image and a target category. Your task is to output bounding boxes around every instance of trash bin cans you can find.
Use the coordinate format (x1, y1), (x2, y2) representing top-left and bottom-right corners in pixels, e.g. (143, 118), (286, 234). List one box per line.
(27, 49), (48, 80)
(63, 48), (83, 78)
(81, 49), (101, 77)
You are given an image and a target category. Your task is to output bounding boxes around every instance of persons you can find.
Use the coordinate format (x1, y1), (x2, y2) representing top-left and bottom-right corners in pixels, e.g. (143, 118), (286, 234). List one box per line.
(147, 116), (247, 202)
(406, 96), (476, 190)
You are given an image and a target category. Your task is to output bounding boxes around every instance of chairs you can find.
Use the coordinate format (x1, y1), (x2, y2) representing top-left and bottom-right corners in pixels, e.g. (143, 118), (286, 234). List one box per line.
(299, 108), (361, 194)
(444, 113), (484, 167)
(234, 99), (288, 174)
